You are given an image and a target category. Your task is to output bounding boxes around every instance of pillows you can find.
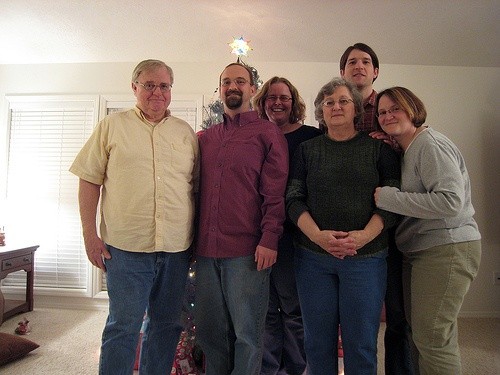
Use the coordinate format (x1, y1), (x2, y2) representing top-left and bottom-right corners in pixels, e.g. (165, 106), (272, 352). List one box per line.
(0, 333), (40, 367)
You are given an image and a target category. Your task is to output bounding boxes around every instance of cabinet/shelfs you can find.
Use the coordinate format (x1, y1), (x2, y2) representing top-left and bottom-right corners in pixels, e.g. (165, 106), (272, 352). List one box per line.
(1, 96), (203, 301)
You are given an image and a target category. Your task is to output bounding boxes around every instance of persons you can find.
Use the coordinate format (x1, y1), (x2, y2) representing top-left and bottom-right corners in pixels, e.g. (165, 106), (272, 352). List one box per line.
(374, 86), (481, 375)
(315, 43), (402, 375)
(69, 59), (201, 375)
(193, 62), (289, 375)
(286, 79), (402, 375)
(250, 75), (318, 375)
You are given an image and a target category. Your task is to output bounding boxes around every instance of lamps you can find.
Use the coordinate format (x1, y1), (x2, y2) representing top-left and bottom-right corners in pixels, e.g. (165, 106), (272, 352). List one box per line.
(229, 36), (251, 63)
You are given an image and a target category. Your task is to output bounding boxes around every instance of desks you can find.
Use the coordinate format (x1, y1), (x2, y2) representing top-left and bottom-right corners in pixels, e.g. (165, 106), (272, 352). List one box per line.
(0, 245), (40, 324)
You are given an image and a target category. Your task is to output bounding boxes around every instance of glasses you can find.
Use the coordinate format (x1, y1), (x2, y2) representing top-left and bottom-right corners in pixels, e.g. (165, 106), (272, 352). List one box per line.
(222, 79), (250, 86)
(135, 81), (172, 92)
(268, 96), (292, 102)
(322, 98), (353, 107)
(378, 107), (402, 114)
